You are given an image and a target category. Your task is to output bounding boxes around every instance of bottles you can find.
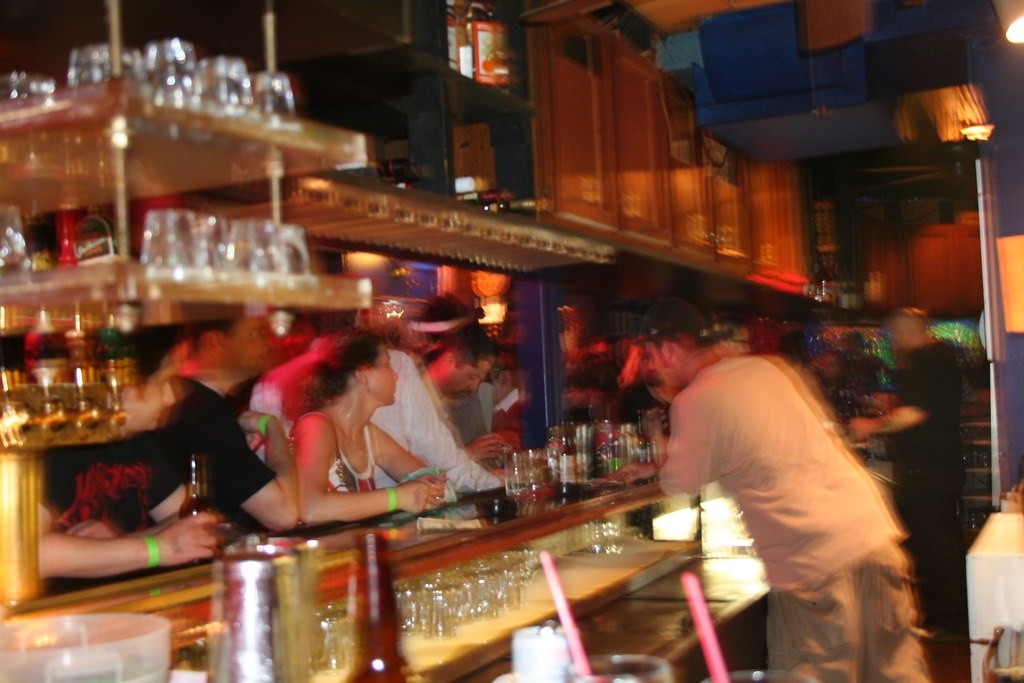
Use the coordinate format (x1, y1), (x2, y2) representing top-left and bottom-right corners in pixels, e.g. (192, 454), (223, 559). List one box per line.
(181, 451), (215, 568)
(445, 0), (511, 95)
(252, 545), (314, 683)
(340, 532), (419, 683)
(375, 159), (433, 188)
(455, 177), (536, 213)
(213, 555), (280, 683)
(552, 410), (656, 499)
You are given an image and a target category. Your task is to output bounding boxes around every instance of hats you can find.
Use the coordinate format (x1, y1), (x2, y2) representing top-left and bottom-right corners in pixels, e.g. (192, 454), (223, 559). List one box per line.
(637, 293), (708, 343)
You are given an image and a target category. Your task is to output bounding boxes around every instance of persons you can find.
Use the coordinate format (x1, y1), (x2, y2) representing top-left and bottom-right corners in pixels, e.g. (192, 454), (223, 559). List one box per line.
(139, 316), (299, 546)
(412, 291), (467, 369)
(847, 305), (967, 642)
(243, 305), (504, 516)
(35, 320), (218, 592)
(490, 315), (884, 439)
(424, 324), (509, 471)
(290, 329), (446, 529)
(627, 294), (933, 682)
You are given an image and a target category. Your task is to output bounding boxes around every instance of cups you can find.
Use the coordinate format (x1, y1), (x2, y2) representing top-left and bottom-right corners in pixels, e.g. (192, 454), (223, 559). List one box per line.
(701, 668), (819, 683)
(525, 448), (545, 496)
(0, 205), (30, 276)
(142, 210), (311, 282)
(568, 653), (674, 683)
(505, 451), (526, 498)
(0, 41), (295, 119)
(314, 543), (535, 674)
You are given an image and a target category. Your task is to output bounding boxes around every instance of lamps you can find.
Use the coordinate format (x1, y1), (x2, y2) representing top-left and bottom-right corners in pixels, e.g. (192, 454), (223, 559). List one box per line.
(991, 1), (1024, 44)
(958, 123), (994, 142)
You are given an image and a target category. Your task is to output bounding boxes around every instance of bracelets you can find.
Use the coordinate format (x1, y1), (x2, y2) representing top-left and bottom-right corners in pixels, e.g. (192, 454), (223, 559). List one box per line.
(384, 486), (396, 514)
(257, 413), (272, 436)
(143, 535), (158, 569)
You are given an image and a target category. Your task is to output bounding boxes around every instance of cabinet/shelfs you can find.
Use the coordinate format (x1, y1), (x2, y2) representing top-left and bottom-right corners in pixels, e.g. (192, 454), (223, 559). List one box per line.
(270, 0), (538, 227)
(522, 13), (815, 298)
(0, 0), (376, 322)
